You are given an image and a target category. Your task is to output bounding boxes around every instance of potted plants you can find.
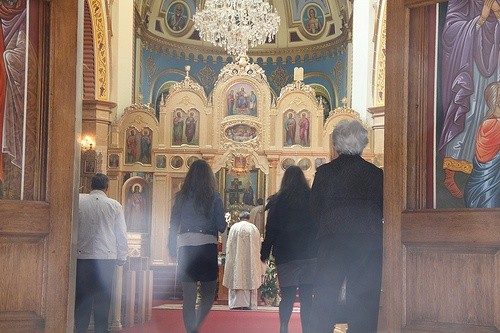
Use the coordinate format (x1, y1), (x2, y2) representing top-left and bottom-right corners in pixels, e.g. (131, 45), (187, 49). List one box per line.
(259, 259), (279, 307)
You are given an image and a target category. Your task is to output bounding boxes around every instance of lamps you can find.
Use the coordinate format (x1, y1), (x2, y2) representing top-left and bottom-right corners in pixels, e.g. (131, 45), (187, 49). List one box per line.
(192, 0), (281, 61)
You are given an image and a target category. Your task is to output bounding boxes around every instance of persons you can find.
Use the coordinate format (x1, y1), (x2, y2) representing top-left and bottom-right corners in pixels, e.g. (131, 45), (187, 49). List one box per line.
(74, 173), (129, 333)
(167, 159), (226, 333)
(260, 116), (384, 333)
(249, 199), (264, 237)
(220, 211), (264, 310)
